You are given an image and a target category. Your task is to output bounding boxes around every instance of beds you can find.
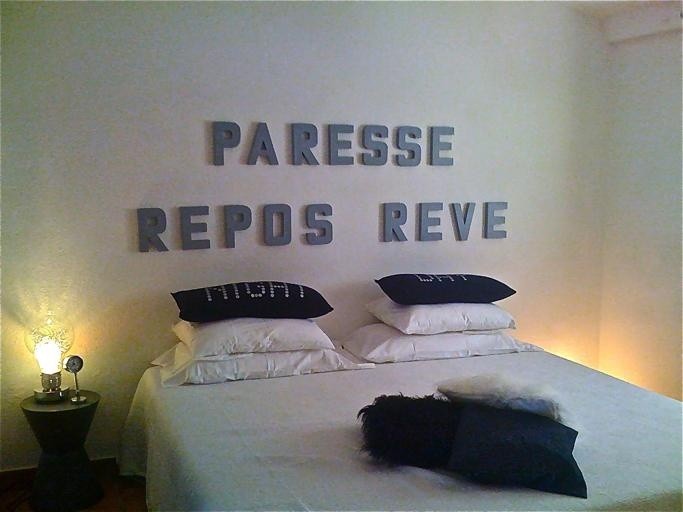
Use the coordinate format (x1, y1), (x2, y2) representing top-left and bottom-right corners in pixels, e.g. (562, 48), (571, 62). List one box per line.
(144, 340), (682, 512)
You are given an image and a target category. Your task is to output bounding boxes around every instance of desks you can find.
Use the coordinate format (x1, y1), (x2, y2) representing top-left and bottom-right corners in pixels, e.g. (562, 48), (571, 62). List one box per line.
(20, 390), (102, 511)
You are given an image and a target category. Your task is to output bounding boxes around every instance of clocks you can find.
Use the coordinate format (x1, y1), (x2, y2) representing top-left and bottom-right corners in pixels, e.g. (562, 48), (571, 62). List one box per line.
(62, 356), (86, 405)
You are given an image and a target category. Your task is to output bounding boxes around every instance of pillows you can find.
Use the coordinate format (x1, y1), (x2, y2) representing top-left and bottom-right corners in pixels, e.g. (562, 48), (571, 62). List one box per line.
(374, 274), (518, 305)
(364, 297), (518, 336)
(354, 375), (587, 500)
(150, 343), (375, 385)
(171, 319), (336, 359)
(338, 323), (545, 363)
(168, 280), (334, 323)
